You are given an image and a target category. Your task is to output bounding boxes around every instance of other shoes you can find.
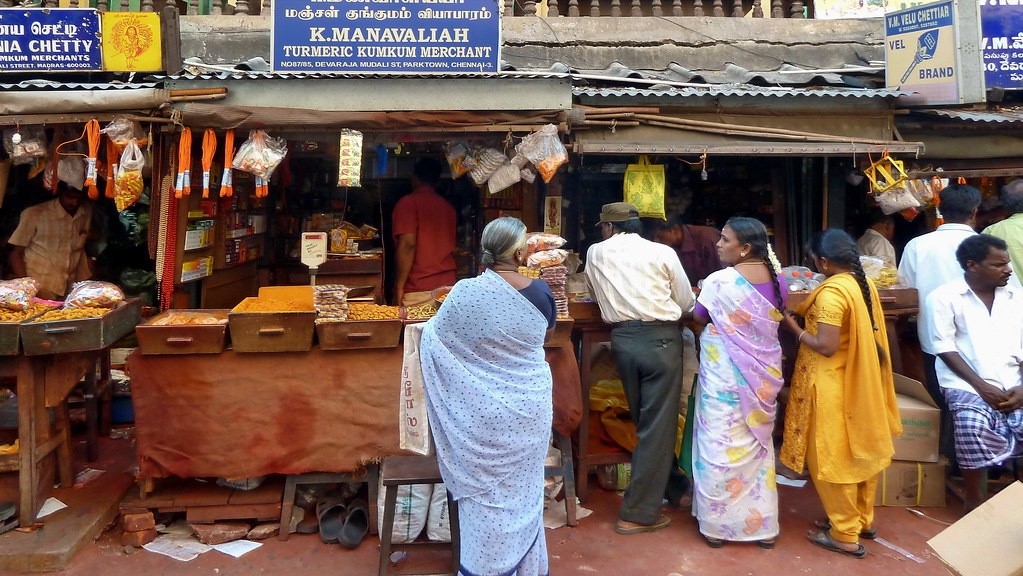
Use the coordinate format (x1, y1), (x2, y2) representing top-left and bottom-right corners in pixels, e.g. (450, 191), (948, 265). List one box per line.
(760, 539), (775, 549)
(677, 502), (691, 511)
(708, 539), (723, 548)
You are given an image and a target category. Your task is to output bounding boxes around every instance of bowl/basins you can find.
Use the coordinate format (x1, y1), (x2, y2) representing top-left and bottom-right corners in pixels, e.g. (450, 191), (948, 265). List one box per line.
(347, 286), (374, 298)
(568, 302), (594, 319)
(786, 292), (812, 311)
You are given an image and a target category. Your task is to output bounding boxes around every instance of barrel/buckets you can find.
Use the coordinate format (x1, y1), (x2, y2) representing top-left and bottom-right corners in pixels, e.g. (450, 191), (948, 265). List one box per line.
(111, 393), (134, 424)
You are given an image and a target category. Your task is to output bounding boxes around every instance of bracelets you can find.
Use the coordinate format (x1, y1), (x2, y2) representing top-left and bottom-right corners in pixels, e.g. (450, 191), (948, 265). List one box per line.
(799, 331), (806, 341)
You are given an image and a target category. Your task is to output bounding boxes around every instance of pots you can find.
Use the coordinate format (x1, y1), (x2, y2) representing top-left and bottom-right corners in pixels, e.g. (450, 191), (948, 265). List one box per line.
(248, 213), (268, 234)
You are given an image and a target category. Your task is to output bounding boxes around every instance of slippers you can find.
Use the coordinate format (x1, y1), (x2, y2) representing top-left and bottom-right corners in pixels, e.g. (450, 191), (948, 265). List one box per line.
(616, 513), (671, 534)
(807, 529), (870, 559)
(816, 516), (878, 538)
(337, 498), (369, 548)
(316, 488), (346, 545)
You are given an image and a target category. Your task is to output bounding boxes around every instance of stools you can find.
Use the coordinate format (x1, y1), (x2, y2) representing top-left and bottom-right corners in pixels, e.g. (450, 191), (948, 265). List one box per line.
(378, 456), (461, 576)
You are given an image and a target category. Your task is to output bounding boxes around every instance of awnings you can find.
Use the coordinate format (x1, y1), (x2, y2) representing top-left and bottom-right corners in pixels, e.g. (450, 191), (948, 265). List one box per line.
(572, 89), (920, 97)
(916, 109), (1023, 123)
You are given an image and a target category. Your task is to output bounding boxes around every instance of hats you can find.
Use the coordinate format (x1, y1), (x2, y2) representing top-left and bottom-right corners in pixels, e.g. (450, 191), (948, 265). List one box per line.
(594, 202), (639, 226)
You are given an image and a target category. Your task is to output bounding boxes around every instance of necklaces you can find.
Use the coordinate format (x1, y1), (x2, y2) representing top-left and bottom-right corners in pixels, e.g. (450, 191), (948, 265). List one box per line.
(496, 271), (515, 272)
(733, 261), (765, 265)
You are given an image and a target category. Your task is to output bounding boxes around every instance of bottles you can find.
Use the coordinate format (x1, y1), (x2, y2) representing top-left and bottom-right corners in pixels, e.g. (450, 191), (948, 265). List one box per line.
(787, 279), (820, 291)
(780, 267), (800, 281)
(812, 272), (828, 284)
(458, 222), (477, 250)
(789, 265), (812, 279)
(697, 279), (704, 289)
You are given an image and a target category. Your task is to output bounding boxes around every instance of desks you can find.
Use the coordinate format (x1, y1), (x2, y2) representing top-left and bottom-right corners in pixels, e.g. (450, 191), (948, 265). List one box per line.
(574, 308), (921, 503)
(0, 345), (113, 527)
(124, 345), (586, 528)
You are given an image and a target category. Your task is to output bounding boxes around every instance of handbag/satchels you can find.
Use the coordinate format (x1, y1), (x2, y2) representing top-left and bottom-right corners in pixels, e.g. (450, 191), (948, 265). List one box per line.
(623, 154), (667, 222)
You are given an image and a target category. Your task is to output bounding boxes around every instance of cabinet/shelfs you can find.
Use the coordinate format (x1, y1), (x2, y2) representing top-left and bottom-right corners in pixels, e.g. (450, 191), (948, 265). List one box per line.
(172, 186), (220, 283)
(214, 177), (264, 269)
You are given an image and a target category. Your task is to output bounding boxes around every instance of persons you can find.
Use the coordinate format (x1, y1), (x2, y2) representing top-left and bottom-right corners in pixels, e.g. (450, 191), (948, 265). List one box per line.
(646, 214), (732, 287)
(392, 159), (457, 306)
(778, 229), (903, 559)
(584, 202), (698, 535)
(8, 179), (93, 301)
(419, 217), (556, 576)
(979, 178), (1023, 288)
(898, 183), (982, 454)
(692, 217), (787, 548)
(855, 208), (898, 269)
(926, 234), (1023, 507)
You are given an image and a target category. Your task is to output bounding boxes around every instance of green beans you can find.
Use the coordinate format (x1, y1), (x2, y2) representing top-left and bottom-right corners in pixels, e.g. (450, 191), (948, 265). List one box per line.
(407, 304), (439, 319)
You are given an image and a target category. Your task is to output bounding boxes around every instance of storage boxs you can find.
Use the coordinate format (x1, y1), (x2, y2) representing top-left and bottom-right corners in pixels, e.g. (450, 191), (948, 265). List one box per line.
(181, 200), (217, 282)
(926, 480), (1023, 576)
(136, 298), (438, 355)
(0, 427), (56, 517)
(312, 214), (333, 230)
(873, 372), (949, 507)
(226, 179), (263, 238)
(0, 298), (141, 356)
